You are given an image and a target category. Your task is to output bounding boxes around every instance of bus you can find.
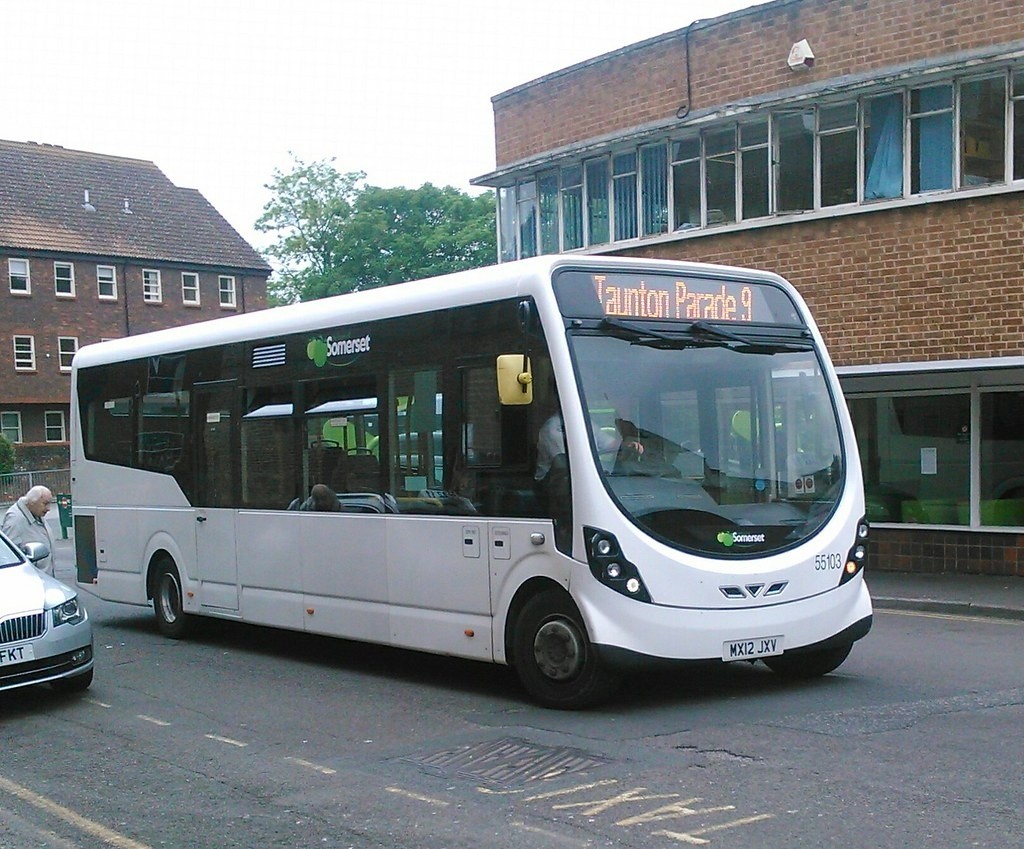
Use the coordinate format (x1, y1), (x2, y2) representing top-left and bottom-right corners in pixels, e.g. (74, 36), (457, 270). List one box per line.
(68, 250), (873, 709)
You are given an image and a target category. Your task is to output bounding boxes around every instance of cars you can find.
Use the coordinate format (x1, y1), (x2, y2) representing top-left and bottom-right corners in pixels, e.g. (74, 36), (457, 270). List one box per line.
(0, 529), (93, 693)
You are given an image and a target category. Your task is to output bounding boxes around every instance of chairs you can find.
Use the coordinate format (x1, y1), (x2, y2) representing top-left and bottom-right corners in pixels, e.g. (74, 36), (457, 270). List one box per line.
(309, 440), (342, 489)
(866, 495), (1024, 527)
(345, 447), (378, 491)
(707, 209), (727, 224)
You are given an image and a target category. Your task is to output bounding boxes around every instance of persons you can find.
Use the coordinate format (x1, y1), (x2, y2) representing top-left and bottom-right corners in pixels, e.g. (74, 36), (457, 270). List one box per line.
(536, 381), (642, 498)
(2, 485), (56, 578)
(308, 484), (345, 514)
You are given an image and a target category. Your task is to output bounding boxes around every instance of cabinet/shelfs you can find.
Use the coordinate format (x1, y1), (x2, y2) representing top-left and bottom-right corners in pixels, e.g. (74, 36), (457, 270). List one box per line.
(961, 119), (1004, 186)
